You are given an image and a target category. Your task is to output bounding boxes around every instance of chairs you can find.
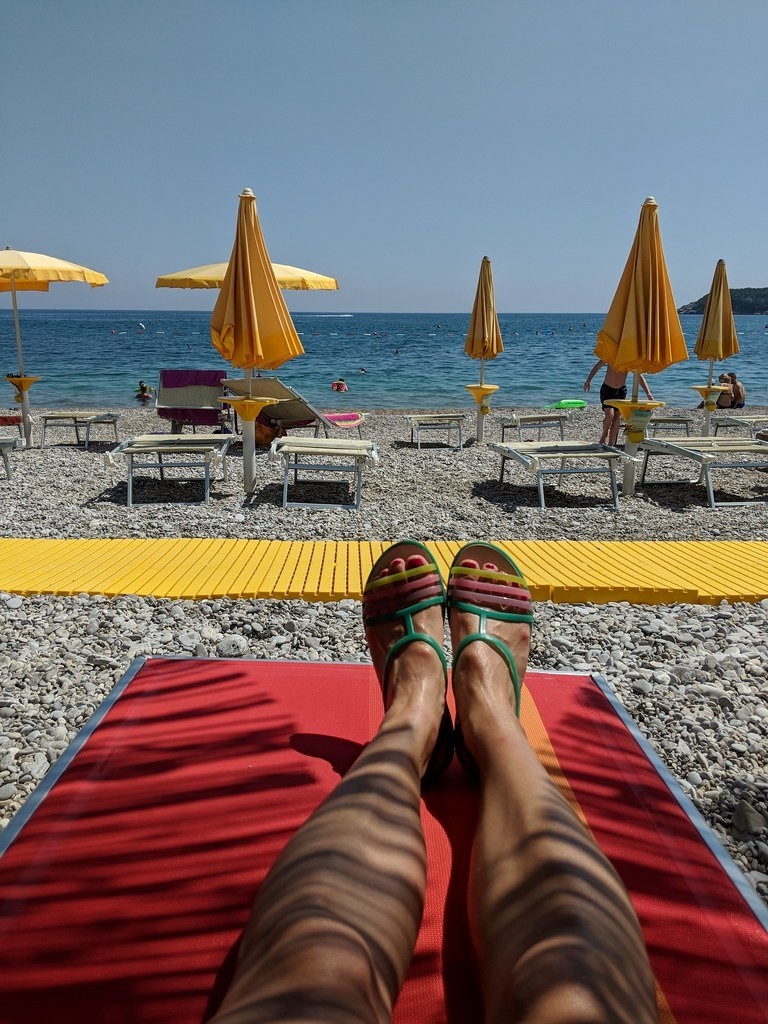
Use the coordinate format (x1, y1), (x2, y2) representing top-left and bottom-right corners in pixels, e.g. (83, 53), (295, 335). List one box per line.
(544, 399), (588, 421)
(0, 438), (18, 480)
(486, 440), (643, 511)
(39, 411), (121, 450)
(499, 414), (567, 442)
(616, 416), (694, 437)
(710, 415), (768, 439)
(155, 370), (243, 436)
(121, 434), (235, 505)
(0, 653), (768, 1024)
(402, 413), (467, 450)
(0, 416), (24, 438)
(220, 376), (365, 440)
(622, 435), (768, 509)
(268, 435), (376, 510)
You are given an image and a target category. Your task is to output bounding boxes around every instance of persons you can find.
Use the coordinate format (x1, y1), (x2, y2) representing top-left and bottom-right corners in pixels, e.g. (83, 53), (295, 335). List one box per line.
(727, 372), (745, 408)
(331, 377), (348, 392)
(200, 536), (676, 1024)
(697, 372), (735, 409)
(583, 359), (655, 445)
(134, 384), (153, 397)
(132, 380), (155, 391)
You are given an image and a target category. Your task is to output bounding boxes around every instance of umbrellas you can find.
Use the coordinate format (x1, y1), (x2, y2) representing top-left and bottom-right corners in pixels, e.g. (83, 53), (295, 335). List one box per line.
(593, 195), (692, 399)
(464, 255), (505, 385)
(209, 186), (305, 398)
(0, 244), (111, 376)
(155, 261), (340, 290)
(693, 257), (740, 387)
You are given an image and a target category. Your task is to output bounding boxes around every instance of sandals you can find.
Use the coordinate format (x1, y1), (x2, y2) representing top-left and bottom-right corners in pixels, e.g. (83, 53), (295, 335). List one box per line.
(443, 542), (535, 779)
(360, 540), (455, 791)
